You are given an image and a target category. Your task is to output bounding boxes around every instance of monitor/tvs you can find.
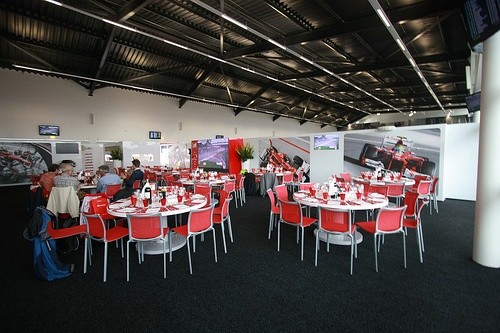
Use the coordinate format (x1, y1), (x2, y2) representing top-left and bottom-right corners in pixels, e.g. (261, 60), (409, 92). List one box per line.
(461, 0), (500, 46)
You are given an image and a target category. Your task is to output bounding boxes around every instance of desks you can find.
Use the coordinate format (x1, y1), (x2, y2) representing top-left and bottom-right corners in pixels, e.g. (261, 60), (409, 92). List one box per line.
(350, 177), (416, 187)
(254, 171), (295, 176)
(107, 192), (207, 255)
(182, 179), (227, 185)
(293, 187), (389, 246)
(78, 183), (98, 189)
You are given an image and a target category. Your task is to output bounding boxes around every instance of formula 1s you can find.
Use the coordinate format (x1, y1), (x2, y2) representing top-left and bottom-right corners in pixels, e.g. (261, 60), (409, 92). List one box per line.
(359, 136), (432, 174)
(262, 143), (306, 181)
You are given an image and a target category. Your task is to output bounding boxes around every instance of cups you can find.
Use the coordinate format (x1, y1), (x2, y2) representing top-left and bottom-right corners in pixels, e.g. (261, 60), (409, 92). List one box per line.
(188, 170), (218, 182)
(311, 186), (362, 201)
(131, 186), (189, 208)
(361, 172), (402, 182)
(259, 167), (283, 173)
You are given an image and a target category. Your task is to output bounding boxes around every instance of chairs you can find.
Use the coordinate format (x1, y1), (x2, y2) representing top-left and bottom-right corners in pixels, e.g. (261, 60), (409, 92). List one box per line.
(23, 167), (439, 282)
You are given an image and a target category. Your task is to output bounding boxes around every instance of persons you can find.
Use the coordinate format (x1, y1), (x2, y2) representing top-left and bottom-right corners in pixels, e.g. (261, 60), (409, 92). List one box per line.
(52, 159), (85, 221)
(39, 164), (61, 202)
(113, 159), (146, 202)
(95, 164), (122, 195)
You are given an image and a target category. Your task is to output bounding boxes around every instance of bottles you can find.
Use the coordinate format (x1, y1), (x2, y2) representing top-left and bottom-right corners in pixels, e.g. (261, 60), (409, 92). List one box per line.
(145, 178), (152, 205)
(157, 177), (166, 202)
